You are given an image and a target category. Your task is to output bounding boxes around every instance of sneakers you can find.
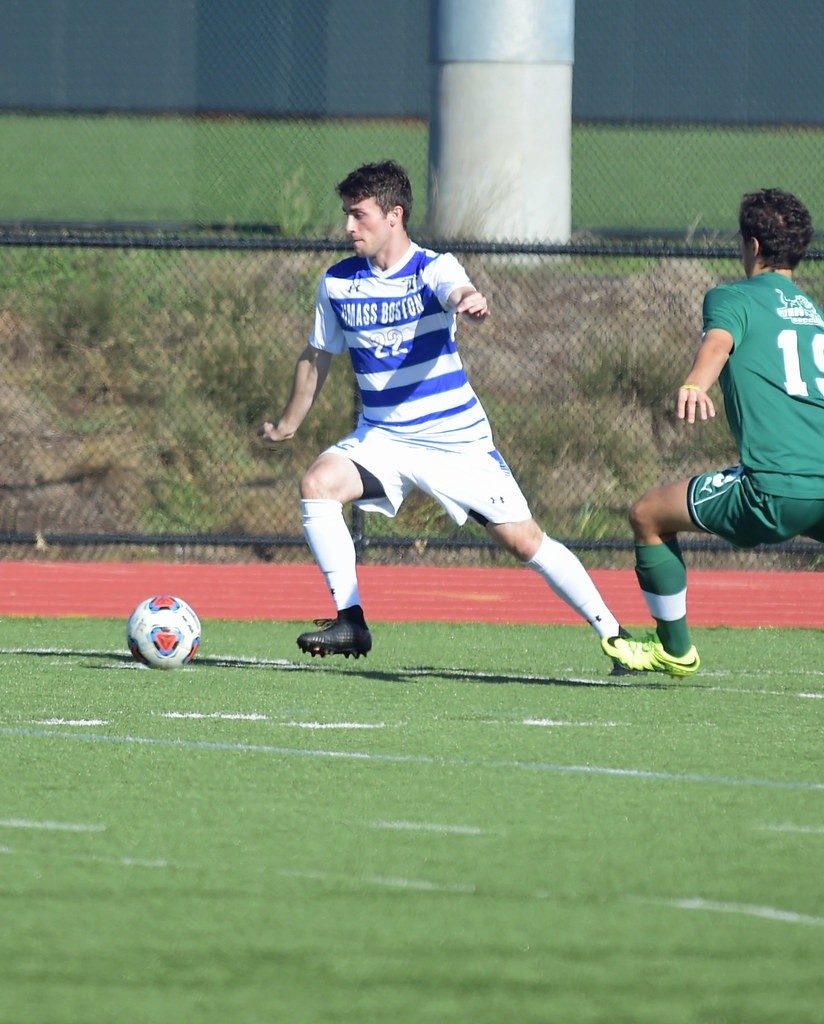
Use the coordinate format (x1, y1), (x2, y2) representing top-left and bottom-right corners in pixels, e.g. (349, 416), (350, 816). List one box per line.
(611, 629), (633, 675)
(600, 630), (699, 678)
(296, 618), (373, 659)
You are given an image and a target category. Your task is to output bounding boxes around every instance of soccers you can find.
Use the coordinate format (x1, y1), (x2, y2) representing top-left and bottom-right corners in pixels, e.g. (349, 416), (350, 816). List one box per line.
(125, 594), (202, 672)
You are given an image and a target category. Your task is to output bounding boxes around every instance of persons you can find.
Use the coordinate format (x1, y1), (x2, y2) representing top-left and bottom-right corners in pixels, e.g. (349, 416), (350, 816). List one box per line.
(255, 159), (640, 672)
(595, 186), (824, 679)
